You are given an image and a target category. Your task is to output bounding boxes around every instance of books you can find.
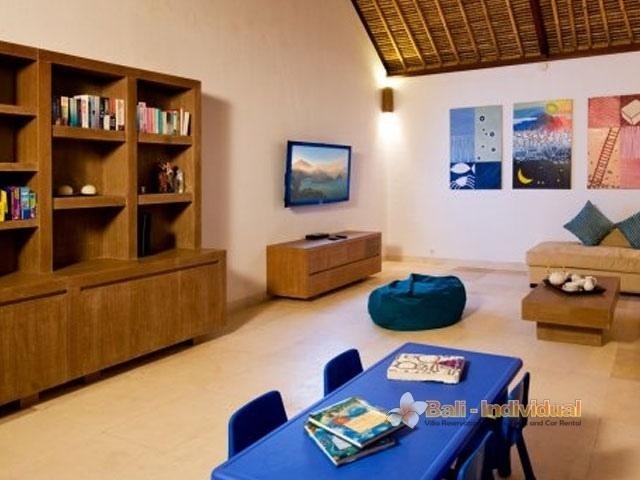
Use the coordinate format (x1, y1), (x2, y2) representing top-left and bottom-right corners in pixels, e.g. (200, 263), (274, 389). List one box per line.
(0, 188), (8, 222)
(9, 186), (21, 221)
(52, 91), (191, 138)
(302, 419), (399, 467)
(29, 192), (36, 218)
(306, 395), (409, 448)
(386, 351), (468, 387)
(21, 186), (31, 221)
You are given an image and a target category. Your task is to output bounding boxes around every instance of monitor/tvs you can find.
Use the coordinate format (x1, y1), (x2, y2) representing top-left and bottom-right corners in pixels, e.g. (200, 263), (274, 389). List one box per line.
(285, 140), (351, 207)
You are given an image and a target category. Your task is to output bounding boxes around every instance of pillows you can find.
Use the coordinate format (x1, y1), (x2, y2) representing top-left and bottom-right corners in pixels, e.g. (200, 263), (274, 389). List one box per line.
(564, 200), (617, 246)
(616, 211), (640, 249)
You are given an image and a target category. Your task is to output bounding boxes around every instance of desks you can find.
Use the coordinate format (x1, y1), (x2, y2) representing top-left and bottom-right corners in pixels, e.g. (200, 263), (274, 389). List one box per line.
(211, 342), (522, 478)
(265, 229), (381, 300)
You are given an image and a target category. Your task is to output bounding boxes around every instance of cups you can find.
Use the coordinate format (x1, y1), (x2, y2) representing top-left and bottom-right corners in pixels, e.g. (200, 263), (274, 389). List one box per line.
(549, 267), (597, 294)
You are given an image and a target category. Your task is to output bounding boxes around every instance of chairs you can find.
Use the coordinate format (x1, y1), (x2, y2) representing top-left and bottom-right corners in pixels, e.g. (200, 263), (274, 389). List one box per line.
(457, 427), (496, 478)
(499, 373), (537, 478)
(229, 391), (288, 457)
(324, 348), (362, 395)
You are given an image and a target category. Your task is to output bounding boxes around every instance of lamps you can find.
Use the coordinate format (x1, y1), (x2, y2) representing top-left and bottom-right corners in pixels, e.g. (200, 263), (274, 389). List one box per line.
(381, 88), (394, 113)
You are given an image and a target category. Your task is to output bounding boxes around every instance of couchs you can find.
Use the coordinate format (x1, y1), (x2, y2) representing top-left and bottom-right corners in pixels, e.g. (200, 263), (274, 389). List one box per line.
(525, 230), (640, 294)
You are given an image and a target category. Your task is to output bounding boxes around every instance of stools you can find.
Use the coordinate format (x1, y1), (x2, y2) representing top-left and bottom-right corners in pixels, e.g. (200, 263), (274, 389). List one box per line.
(367, 271), (468, 332)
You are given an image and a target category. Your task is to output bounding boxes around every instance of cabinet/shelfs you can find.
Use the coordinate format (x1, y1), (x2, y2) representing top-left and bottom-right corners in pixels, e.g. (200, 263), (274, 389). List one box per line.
(0, 41), (227, 409)
